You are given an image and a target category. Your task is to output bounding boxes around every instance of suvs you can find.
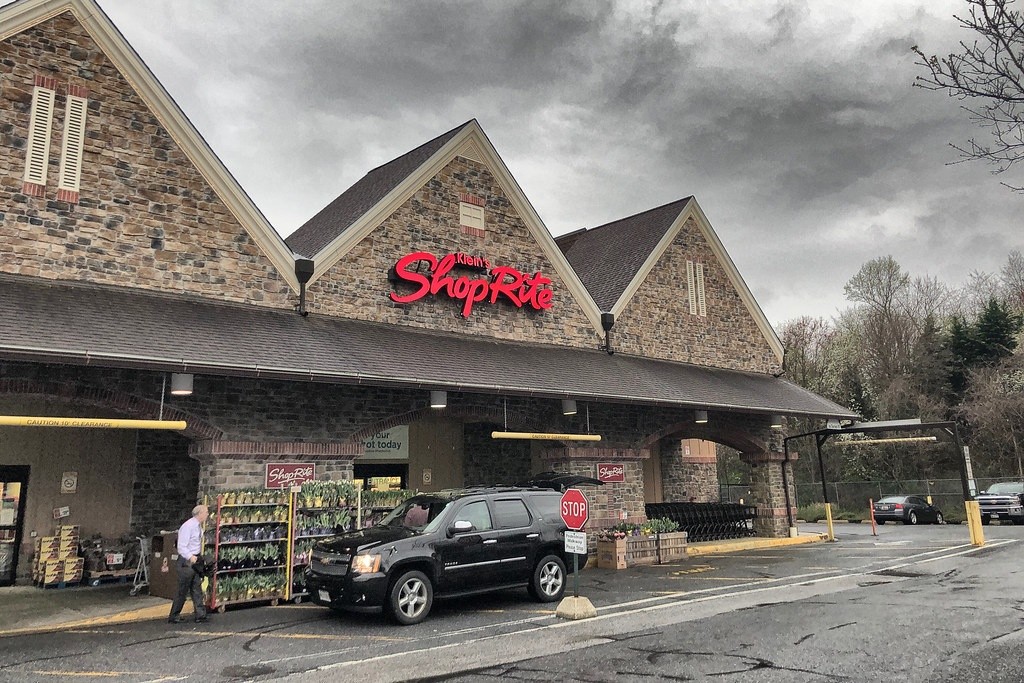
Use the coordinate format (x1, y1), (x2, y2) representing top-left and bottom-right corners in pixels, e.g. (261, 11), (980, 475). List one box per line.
(307, 485), (589, 626)
(974, 482), (1024, 526)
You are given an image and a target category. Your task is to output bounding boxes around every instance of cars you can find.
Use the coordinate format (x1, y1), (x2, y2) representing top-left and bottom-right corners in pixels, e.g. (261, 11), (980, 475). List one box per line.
(873, 496), (943, 525)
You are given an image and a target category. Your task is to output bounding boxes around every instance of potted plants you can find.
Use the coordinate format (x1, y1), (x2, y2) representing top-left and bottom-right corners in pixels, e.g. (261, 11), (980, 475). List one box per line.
(204, 482), (289, 603)
(361, 490), (415, 527)
(645, 517), (688, 564)
(292, 510), (352, 594)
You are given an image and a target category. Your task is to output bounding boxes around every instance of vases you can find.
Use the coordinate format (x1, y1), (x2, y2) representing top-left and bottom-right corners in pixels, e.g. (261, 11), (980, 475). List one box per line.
(298, 496), (358, 508)
(626, 534), (659, 567)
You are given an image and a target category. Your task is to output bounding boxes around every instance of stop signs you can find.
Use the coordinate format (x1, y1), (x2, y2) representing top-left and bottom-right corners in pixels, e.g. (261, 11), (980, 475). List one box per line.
(559, 488), (588, 530)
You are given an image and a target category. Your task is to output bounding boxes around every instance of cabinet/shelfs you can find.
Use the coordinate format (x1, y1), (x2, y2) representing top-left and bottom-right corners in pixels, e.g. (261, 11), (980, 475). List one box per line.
(201, 492), (292, 611)
(290, 482), (361, 602)
(150, 533), (179, 599)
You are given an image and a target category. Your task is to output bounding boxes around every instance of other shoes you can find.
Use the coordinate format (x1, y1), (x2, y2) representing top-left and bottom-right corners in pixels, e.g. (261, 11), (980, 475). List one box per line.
(195, 617), (212, 623)
(168, 618), (185, 623)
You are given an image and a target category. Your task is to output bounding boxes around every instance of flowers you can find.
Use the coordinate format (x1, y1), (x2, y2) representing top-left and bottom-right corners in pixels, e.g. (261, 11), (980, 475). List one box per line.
(603, 519), (652, 541)
(300, 478), (357, 498)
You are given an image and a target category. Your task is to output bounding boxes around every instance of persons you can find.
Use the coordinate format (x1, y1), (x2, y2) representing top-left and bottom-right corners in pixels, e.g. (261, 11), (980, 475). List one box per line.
(168, 505), (213, 624)
(405, 491), (429, 527)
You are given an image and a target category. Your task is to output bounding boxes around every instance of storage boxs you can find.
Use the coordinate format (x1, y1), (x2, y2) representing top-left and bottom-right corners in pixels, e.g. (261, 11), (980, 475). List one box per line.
(598, 540), (626, 569)
(32, 524), (84, 583)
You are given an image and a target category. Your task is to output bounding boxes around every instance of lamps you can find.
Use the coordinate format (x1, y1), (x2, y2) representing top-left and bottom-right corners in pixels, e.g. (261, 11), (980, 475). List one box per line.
(171, 373), (193, 395)
(694, 410), (708, 423)
(562, 400), (577, 415)
(770, 415), (782, 428)
(429, 391), (447, 407)
